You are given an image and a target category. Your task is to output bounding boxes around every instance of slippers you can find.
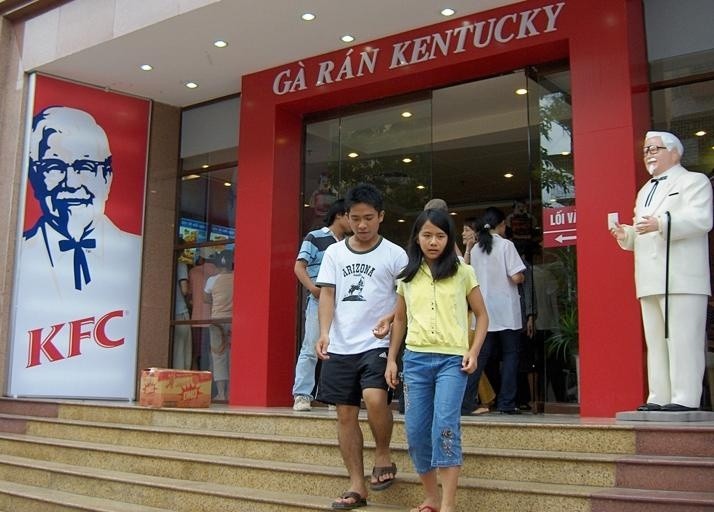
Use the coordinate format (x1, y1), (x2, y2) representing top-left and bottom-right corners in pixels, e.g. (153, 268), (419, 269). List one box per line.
(370, 462), (396, 490)
(332, 492), (367, 508)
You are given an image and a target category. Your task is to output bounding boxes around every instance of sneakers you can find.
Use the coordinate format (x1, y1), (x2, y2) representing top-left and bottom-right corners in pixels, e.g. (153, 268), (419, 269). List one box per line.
(293, 396), (312, 412)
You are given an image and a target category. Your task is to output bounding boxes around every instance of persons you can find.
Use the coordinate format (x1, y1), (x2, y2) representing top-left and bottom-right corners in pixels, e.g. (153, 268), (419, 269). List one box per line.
(384, 210), (489, 512)
(10, 106), (142, 399)
(292, 198), (352, 411)
(610, 130), (713, 412)
(315, 184), (410, 510)
(399, 199), (568, 417)
(173, 238), (234, 401)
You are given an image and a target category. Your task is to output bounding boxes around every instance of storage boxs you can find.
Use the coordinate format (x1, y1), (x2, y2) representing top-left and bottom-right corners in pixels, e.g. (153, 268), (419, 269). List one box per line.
(135, 364), (213, 411)
